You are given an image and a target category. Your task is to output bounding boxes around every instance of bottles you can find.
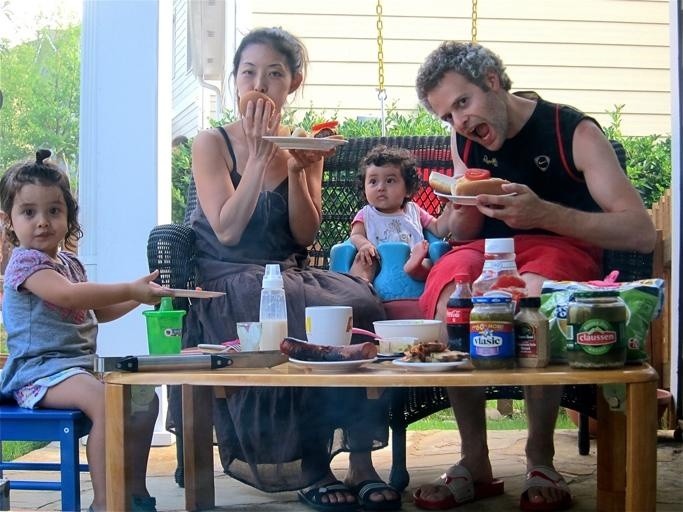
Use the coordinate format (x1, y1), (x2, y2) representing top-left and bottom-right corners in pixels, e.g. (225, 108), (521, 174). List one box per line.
(515, 297), (550, 367)
(446, 274), (471, 351)
(471, 237), (519, 297)
(260, 265), (287, 351)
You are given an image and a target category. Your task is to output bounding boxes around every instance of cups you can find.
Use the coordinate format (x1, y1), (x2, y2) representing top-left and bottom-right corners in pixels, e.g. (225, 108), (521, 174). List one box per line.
(305, 306), (353, 347)
(236, 323), (259, 351)
(142, 297), (185, 356)
(375, 337), (417, 353)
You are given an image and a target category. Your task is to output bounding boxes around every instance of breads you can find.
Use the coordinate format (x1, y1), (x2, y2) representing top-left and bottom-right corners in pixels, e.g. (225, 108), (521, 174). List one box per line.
(428, 171), (457, 195)
(430, 351), (469, 362)
(450, 174), (513, 195)
(239, 91), (276, 122)
(277, 124), (306, 138)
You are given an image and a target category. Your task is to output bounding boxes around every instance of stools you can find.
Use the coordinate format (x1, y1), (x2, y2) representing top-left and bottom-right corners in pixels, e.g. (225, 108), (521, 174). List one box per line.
(1, 407), (91, 511)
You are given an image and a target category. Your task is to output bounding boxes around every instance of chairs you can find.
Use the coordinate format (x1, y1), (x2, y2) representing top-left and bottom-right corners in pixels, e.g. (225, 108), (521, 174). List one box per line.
(330, 227), (452, 301)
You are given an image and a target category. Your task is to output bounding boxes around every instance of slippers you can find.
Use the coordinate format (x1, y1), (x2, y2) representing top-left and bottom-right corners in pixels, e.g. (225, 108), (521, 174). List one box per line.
(343, 465), (402, 511)
(296, 473), (355, 511)
(410, 462), (505, 511)
(518, 465), (573, 511)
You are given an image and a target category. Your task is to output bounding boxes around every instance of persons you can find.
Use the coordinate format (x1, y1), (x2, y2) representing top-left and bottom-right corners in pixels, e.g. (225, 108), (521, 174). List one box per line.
(414, 38), (656, 504)
(185, 26), (402, 506)
(350, 144), (458, 283)
(0, 148), (174, 511)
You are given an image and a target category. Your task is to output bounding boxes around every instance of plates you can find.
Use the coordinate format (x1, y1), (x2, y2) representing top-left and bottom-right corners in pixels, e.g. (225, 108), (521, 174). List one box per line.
(290, 357), (378, 370)
(434, 189), (517, 205)
(393, 359), (467, 372)
(262, 135), (348, 151)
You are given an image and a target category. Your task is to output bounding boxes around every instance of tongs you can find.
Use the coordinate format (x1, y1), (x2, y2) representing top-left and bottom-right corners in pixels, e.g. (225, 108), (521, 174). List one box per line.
(94, 353), (288, 372)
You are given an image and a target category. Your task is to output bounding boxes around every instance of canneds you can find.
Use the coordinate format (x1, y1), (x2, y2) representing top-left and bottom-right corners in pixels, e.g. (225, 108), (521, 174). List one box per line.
(469, 296), (515, 371)
(566, 291), (629, 370)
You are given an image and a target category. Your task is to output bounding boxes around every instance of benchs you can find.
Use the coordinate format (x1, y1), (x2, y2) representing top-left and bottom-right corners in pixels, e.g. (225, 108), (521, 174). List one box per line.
(146, 137), (654, 491)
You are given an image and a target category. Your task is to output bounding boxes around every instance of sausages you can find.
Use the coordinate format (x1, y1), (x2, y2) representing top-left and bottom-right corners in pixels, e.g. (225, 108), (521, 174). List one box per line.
(280, 337), (377, 361)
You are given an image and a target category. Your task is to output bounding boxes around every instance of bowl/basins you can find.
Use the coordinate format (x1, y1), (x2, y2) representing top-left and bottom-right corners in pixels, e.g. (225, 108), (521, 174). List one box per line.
(373, 320), (442, 343)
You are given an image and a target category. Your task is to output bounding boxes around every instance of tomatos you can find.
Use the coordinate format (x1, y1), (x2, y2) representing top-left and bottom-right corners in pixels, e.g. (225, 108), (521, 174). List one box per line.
(312, 121), (339, 131)
(465, 168), (491, 181)
